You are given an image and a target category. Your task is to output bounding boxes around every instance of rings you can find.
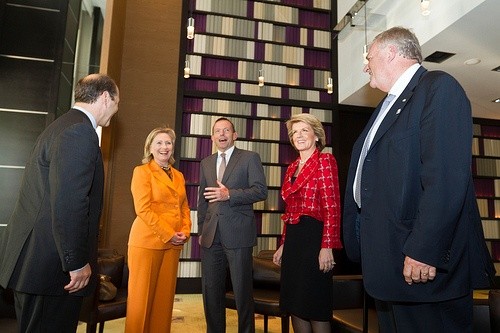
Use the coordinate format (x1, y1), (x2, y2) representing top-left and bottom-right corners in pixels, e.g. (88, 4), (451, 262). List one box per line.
(331, 262), (336, 265)
(421, 273), (428, 276)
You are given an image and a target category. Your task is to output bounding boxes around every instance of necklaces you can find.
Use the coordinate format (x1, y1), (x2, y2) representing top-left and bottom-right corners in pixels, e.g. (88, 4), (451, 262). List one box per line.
(300, 150), (315, 164)
(160, 165), (170, 170)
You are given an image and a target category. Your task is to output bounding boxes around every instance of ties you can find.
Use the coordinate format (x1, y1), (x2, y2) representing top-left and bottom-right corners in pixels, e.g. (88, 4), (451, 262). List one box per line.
(353, 94), (396, 208)
(217, 153), (226, 182)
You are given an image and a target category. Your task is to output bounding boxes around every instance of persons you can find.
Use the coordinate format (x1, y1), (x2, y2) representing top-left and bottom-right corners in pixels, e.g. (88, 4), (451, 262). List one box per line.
(195, 118), (268, 333)
(342, 26), (494, 333)
(125, 129), (191, 333)
(273, 114), (344, 333)
(0, 74), (120, 333)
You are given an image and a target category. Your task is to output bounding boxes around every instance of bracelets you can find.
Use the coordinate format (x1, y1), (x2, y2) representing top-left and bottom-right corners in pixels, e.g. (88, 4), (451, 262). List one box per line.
(228, 194), (230, 200)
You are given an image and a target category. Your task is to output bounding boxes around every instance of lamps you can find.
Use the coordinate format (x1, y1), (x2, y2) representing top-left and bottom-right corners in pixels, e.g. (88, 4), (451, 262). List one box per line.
(421, 0), (431, 15)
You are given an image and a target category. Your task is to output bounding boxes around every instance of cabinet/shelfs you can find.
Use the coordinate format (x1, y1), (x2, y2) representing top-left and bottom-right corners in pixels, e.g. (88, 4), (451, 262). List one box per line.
(173, 0), (339, 294)
(472, 116), (500, 289)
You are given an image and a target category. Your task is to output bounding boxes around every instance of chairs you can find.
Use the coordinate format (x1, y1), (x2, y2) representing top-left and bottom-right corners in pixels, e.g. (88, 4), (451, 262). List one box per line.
(330, 276), (379, 333)
(225, 251), (289, 333)
(79, 249), (128, 333)
